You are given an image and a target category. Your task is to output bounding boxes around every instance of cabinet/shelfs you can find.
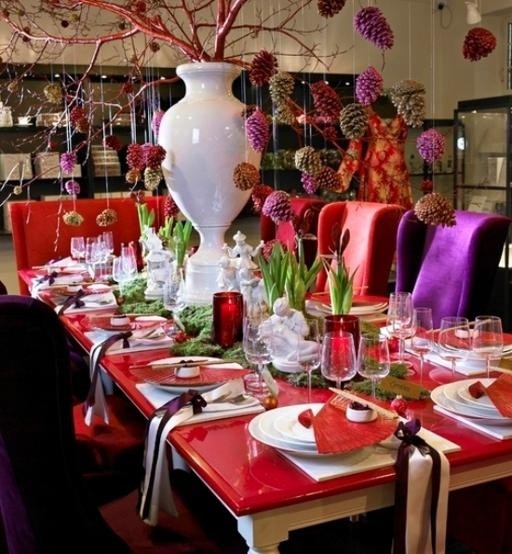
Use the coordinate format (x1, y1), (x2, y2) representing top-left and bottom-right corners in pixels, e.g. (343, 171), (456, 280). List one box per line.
(453, 94), (510, 269)
(0, 61), (359, 233)
(402, 118), (466, 209)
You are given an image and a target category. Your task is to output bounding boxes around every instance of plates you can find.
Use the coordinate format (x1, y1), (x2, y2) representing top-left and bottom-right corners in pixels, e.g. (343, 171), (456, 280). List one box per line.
(430, 377), (510, 421)
(248, 406), (323, 458)
(146, 356), (243, 394)
(316, 302), (389, 316)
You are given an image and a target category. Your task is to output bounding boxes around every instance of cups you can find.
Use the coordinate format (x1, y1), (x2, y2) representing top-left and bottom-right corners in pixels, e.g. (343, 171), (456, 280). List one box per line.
(296, 320), (323, 404)
(212, 291), (243, 348)
(113, 259), (121, 295)
(385, 291), (415, 379)
(356, 333), (391, 397)
(321, 332), (357, 387)
(242, 314), (275, 398)
(471, 317), (504, 378)
(324, 315), (361, 388)
(437, 317), (471, 380)
(409, 306), (434, 386)
(121, 247), (136, 278)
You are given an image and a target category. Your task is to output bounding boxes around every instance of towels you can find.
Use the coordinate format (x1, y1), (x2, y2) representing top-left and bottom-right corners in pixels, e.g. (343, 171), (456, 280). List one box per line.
(136, 377), (246, 527)
(31, 272), (84, 299)
(57, 290), (113, 328)
(379, 418), (450, 554)
(82, 323), (159, 427)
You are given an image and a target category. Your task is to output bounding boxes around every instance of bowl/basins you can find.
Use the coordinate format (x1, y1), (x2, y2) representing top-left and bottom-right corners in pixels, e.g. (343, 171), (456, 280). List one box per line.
(111, 317), (130, 326)
(173, 366), (200, 379)
(345, 403), (377, 422)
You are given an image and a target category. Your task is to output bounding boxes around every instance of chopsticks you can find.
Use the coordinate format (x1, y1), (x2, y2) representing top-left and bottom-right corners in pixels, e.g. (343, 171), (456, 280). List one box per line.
(151, 356), (233, 370)
(328, 387), (398, 421)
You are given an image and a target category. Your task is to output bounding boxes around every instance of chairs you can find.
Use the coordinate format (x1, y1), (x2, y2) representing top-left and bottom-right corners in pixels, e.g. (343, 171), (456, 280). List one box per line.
(9, 196), (157, 295)
(260, 197), (325, 263)
(395, 209), (512, 329)
(313, 202), (405, 296)
(0, 280), (144, 554)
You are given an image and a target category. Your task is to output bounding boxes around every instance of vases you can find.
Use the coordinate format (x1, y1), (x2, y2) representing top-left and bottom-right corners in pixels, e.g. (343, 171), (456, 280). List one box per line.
(156, 63), (262, 303)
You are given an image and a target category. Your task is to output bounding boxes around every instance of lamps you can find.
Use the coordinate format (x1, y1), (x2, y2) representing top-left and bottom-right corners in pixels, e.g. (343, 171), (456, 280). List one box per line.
(464, 0), (482, 27)
